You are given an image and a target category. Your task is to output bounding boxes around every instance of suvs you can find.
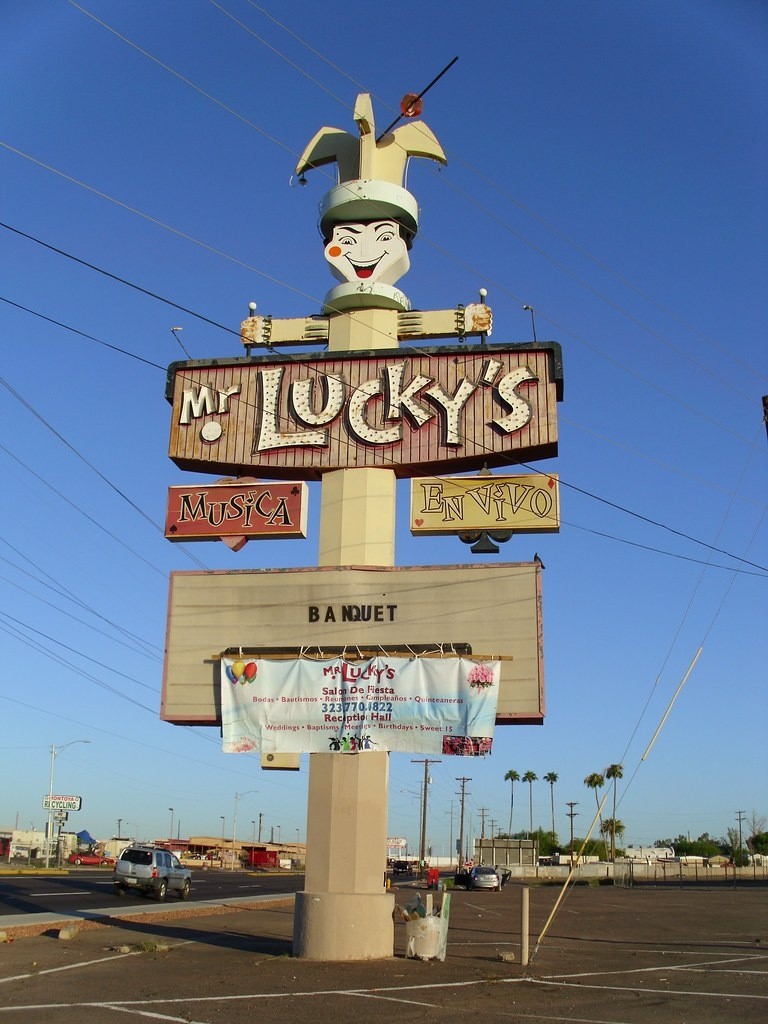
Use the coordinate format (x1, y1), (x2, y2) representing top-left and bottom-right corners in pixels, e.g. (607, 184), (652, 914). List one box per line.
(113, 843), (192, 901)
(393, 860), (412, 876)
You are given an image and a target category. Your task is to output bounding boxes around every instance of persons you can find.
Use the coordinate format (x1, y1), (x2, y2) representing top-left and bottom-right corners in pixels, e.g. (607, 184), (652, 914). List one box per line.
(469, 859), (475, 867)
(419, 856), (427, 881)
(494, 864), (503, 891)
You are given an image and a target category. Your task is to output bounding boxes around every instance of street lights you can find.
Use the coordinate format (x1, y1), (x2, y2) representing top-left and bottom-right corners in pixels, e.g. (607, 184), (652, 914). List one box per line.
(46, 740), (92, 868)
(401, 789), (422, 873)
(231, 790), (261, 871)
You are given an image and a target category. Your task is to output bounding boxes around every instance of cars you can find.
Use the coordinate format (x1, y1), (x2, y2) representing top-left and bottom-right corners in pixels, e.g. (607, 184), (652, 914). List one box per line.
(454, 866), (512, 892)
(69, 851), (115, 865)
